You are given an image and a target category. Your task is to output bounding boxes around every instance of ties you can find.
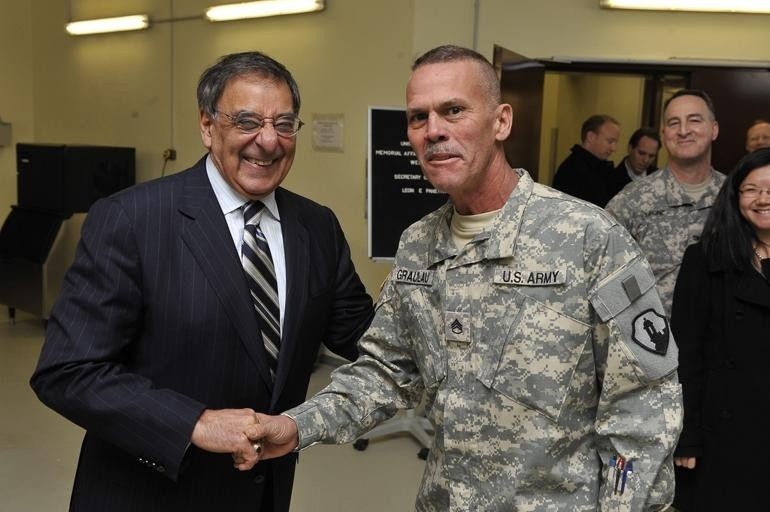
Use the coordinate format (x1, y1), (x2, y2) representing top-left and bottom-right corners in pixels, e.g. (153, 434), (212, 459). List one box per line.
(239, 199), (280, 380)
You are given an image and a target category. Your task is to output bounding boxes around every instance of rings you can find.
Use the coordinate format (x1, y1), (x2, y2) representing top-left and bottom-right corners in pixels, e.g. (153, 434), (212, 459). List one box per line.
(251, 441), (263, 459)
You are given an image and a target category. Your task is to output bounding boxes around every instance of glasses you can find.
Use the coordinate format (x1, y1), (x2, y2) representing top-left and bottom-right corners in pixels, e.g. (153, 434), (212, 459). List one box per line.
(214, 107), (303, 137)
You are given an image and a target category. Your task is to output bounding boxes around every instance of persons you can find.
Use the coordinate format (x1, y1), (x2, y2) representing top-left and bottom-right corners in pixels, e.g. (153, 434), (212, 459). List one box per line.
(605, 124), (662, 209)
(231, 44), (685, 511)
(552, 111), (621, 212)
(668, 145), (770, 509)
(743, 117), (770, 156)
(28, 50), (375, 512)
(601, 87), (732, 332)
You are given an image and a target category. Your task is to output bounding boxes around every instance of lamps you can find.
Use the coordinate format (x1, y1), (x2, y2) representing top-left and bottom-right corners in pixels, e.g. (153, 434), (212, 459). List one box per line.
(65, 12), (150, 35)
(597, 0), (769, 15)
(204, 0), (326, 23)
